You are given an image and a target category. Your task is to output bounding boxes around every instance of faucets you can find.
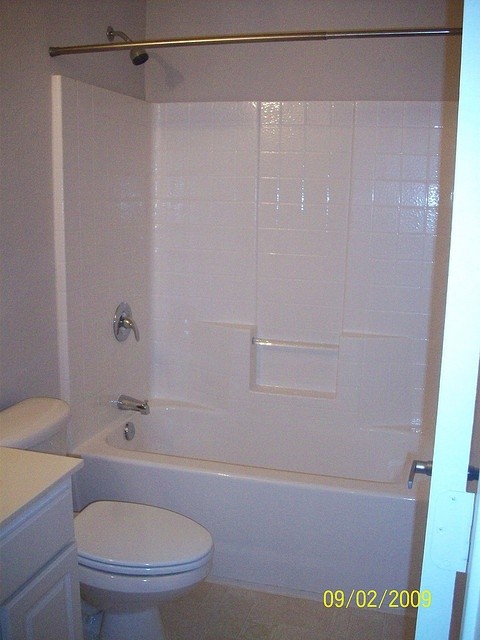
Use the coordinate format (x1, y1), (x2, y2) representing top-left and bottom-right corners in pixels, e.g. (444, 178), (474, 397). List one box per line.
(119, 395), (151, 416)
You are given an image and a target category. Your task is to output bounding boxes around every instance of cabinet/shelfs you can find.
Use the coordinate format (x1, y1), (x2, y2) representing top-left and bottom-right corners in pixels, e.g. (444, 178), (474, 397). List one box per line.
(1, 445), (85, 640)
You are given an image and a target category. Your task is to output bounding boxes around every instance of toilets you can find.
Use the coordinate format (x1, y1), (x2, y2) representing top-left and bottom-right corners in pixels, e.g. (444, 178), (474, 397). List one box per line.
(0, 396), (213, 640)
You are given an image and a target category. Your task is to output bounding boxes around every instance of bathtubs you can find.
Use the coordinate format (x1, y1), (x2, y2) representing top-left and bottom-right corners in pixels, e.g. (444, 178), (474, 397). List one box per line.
(75, 396), (429, 618)
